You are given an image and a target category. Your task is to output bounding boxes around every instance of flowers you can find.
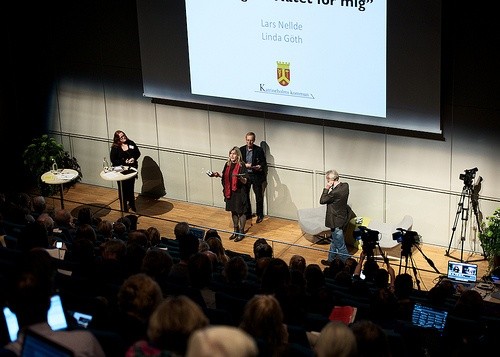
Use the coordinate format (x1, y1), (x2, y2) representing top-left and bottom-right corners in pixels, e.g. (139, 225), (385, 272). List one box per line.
(22, 134), (64, 170)
(477, 207), (500, 261)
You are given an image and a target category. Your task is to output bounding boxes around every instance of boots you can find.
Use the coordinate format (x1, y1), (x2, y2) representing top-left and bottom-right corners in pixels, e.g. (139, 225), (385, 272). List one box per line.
(229, 225), (240, 240)
(234, 228), (245, 242)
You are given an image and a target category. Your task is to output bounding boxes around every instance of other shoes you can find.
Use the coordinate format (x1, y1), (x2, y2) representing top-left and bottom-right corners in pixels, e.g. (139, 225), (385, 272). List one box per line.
(246, 213), (252, 220)
(256, 215), (263, 223)
(128, 201), (137, 212)
(124, 206), (128, 213)
(321, 260), (330, 266)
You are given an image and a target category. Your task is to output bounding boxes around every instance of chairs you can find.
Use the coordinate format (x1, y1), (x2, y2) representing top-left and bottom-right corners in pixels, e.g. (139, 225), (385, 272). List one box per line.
(0, 200), (500, 357)
(297, 206), (333, 246)
(360, 214), (414, 261)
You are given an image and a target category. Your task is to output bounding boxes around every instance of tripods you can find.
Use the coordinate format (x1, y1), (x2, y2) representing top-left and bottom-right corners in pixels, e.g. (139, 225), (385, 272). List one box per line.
(446, 184), (490, 263)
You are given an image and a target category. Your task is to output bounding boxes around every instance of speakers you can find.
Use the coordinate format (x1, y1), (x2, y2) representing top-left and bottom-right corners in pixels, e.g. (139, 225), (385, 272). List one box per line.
(190, 228), (205, 238)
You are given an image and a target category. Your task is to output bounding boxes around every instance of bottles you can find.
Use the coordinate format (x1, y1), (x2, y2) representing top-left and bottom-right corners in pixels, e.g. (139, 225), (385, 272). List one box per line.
(51, 159), (58, 175)
(102, 157), (109, 173)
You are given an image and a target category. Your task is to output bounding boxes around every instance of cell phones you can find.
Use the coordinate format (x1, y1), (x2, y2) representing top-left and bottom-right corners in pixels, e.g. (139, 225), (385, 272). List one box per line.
(56, 241), (62, 248)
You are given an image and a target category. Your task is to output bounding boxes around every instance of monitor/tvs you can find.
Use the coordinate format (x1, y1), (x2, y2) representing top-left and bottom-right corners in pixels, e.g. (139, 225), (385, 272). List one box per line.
(410, 304), (448, 336)
(447, 260), (477, 282)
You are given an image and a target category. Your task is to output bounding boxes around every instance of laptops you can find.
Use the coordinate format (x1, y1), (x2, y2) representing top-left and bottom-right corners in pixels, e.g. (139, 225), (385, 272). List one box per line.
(73, 312), (94, 329)
(19, 331), (75, 357)
(3, 295), (70, 342)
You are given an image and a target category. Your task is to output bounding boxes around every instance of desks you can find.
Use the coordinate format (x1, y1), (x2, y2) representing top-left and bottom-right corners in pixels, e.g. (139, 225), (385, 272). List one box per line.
(27, 319), (103, 357)
(349, 216), (371, 250)
(41, 169), (79, 209)
(100, 166), (139, 225)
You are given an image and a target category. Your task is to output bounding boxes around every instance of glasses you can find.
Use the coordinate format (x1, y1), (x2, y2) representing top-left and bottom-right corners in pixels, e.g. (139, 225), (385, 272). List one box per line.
(120, 134), (125, 140)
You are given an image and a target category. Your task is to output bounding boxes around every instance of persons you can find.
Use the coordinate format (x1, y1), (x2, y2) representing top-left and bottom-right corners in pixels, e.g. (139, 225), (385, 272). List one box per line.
(214, 146), (249, 242)
(110, 130), (141, 213)
(0, 192), (500, 357)
(319, 169), (357, 265)
(240, 132), (268, 223)
